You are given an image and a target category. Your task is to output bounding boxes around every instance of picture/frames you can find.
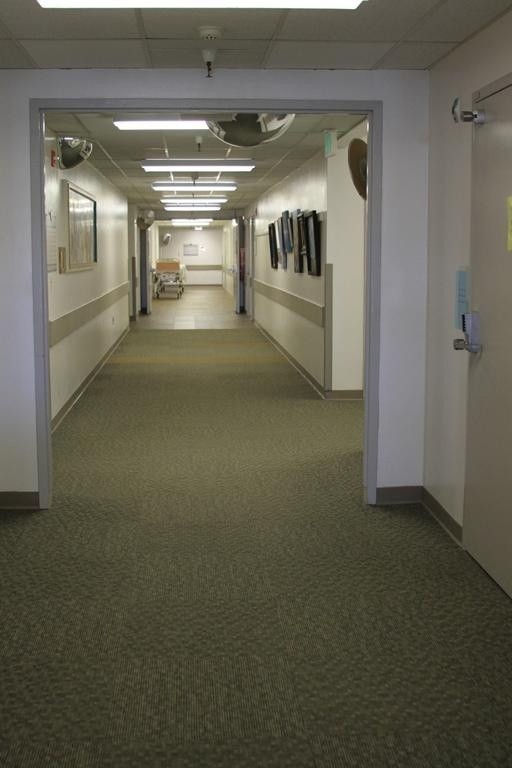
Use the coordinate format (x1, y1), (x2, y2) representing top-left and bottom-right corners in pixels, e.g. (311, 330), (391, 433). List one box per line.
(61, 179), (100, 274)
(267, 207), (321, 277)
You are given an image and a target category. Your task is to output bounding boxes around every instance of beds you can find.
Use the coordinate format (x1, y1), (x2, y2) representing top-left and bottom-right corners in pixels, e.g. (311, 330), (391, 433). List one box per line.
(153, 256), (189, 299)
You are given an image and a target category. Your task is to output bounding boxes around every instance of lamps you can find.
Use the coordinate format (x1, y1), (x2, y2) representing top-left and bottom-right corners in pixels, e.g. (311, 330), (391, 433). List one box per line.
(150, 180), (239, 194)
(159, 194), (229, 227)
(139, 158), (256, 174)
(111, 116), (209, 132)
